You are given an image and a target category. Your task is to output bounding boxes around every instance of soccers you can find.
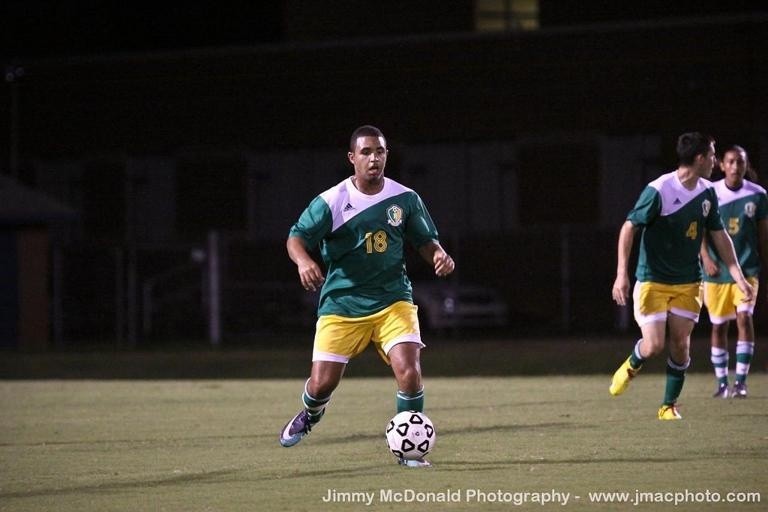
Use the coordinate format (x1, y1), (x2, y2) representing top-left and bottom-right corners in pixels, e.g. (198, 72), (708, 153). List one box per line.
(386, 409), (435, 460)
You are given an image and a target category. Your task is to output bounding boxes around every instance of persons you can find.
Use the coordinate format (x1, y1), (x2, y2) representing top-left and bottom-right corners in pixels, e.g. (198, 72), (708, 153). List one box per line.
(697, 141), (766, 400)
(277, 124), (456, 469)
(607, 129), (753, 417)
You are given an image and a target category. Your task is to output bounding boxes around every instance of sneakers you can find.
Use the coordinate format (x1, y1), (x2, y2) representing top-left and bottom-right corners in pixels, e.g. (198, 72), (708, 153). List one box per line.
(714, 381), (729, 399)
(279, 408), (316, 448)
(608, 356), (643, 396)
(657, 404), (683, 421)
(397, 455), (433, 469)
(732, 384), (747, 398)
